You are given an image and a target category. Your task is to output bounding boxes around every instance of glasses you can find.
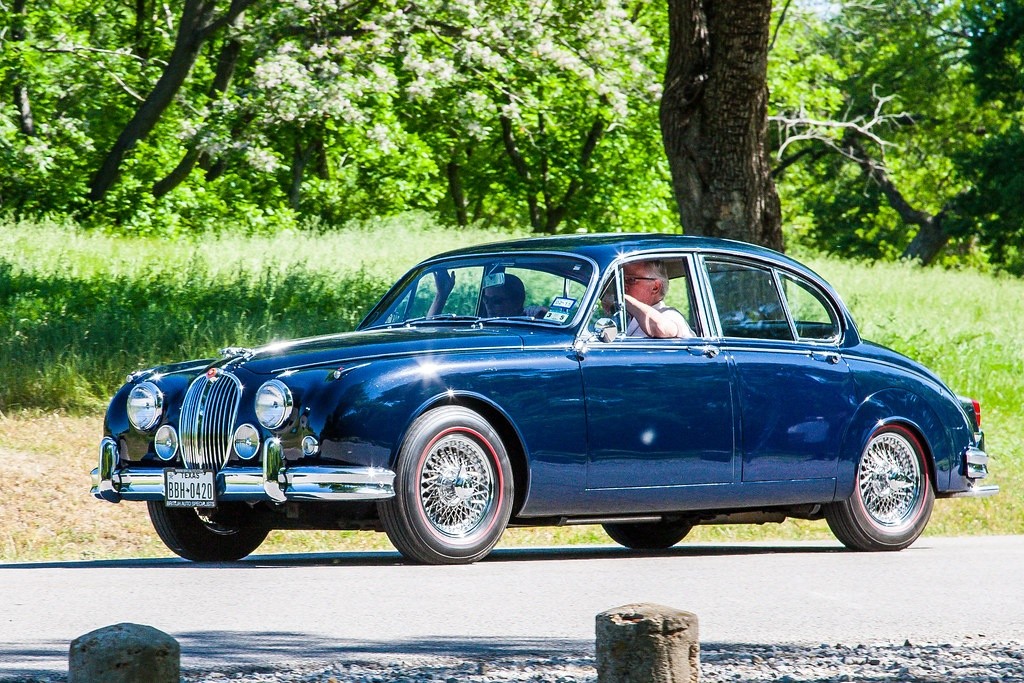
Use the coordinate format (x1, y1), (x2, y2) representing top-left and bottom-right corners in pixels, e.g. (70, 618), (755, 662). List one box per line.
(625, 275), (657, 285)
(482, 295), (510, 304)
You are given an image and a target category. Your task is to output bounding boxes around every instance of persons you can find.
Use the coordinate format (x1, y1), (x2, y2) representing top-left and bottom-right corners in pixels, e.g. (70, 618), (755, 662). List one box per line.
(426, 268), (547, 319)
(601, 260), (696, 339)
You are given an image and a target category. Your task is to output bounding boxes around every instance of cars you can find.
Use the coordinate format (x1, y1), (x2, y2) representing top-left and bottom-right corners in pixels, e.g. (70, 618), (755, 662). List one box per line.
(90, 233), (1000, 565)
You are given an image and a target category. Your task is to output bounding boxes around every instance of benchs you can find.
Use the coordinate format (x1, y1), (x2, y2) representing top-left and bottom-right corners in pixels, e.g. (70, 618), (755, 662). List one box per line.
(690, 320), (833, 341)
